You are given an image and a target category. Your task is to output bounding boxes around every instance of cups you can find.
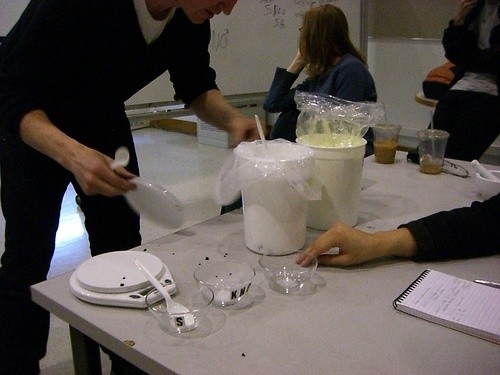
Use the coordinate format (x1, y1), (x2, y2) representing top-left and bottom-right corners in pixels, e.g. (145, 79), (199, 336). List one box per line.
(417, 129), (449, 174)
(235, 142), (313, 255)
(373, 123), (398, 163)
(296, 133), (367, 231)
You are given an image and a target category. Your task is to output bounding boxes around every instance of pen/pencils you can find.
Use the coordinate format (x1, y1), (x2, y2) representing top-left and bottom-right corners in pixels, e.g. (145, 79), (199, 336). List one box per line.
(473, 277), (500, 287)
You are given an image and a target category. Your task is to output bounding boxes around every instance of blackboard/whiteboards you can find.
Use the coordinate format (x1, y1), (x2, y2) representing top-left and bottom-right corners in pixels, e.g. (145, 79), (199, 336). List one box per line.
(0, 0), (370, 117)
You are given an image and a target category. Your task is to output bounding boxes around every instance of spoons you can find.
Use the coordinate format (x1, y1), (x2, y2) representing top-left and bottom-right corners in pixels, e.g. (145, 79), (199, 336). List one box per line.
(136, 260), (195, 327)
(110, 147), (129, 172)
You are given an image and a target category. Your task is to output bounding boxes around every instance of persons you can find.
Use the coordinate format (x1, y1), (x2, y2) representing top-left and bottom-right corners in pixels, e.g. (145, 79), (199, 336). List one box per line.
(295, 191), (500, 267)
(416, 0), (500, 162)
(263, 4), (378, 158)
(0, 0), (264, 375)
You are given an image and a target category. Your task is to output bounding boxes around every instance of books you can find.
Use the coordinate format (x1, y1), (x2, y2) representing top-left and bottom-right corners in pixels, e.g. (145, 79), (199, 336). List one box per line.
(393, 269), (500, 345)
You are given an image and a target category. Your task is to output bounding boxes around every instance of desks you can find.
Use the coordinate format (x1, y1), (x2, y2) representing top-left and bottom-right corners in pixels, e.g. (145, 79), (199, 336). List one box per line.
(30, 151), (500, 375)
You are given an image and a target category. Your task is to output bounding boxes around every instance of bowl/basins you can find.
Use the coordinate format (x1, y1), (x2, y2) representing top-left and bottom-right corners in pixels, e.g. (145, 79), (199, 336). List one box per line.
(146, 282), (214, 333)
(194, 259), (255, 307)
(259, 250), (317, 293)
(475, 170), (500, 200)
